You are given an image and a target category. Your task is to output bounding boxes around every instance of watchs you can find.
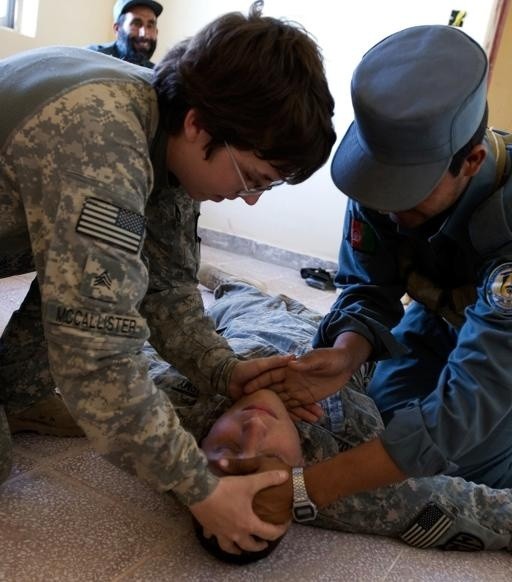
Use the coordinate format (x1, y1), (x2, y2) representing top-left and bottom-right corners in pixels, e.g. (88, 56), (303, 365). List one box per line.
(292, 467), (319, 523)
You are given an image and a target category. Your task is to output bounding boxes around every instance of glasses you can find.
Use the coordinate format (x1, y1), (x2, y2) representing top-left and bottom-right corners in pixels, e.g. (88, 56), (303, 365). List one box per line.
(226, 143), (286, 198)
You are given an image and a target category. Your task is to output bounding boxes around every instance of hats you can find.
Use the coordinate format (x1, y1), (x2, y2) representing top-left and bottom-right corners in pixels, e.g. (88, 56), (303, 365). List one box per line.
(113, 0), (163, 23)
(330, 24), (489, 213)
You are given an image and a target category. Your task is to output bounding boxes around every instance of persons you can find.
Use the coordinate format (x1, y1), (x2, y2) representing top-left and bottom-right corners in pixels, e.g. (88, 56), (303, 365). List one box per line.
(1, 0), (337, 557)
(83, 0), (164, 70)
(0, 258), (511, 567)
(216, 24), (511, 526)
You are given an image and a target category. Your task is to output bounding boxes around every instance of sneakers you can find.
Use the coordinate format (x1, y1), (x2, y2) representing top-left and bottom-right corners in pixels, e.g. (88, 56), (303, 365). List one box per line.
(6, 387), (87, 437)
(197, 264), (267, 294)
